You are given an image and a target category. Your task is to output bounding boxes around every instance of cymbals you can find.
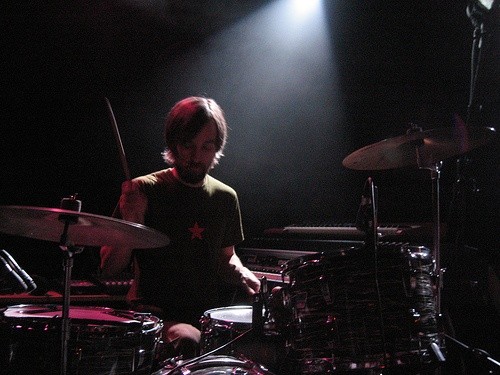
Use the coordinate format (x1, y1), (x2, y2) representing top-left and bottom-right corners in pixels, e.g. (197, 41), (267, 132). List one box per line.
(341, 122), (500, 171)
(0, 194), (168, 250)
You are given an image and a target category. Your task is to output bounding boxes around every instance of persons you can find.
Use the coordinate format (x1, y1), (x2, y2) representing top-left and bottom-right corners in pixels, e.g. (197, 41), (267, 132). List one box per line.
(101, 97), (281, 361)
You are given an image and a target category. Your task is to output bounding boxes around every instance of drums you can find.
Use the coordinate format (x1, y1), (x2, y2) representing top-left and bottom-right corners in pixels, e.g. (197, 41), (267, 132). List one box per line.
(4, 304), (164, 375)
(276, 244), (445, 375)
(152, 353), (273, 375)
(201, 305), (296, 375)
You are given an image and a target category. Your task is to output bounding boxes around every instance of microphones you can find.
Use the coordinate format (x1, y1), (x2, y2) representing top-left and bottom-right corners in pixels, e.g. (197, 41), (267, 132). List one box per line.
(465, 6), (484, 33)
